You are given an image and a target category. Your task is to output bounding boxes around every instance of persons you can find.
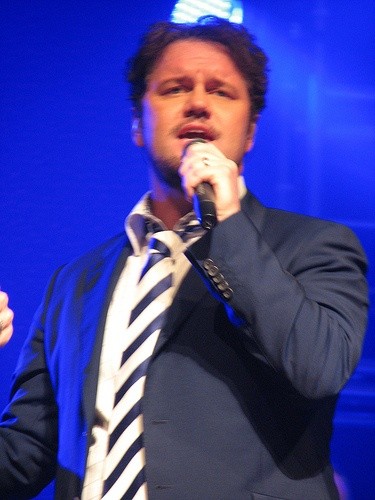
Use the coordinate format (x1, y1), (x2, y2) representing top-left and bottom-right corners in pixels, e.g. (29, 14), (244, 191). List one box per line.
(0, 14), (371, 499)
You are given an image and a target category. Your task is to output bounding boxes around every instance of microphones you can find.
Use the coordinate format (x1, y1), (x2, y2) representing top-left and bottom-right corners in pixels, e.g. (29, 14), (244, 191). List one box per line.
(185, 140), (221, 230)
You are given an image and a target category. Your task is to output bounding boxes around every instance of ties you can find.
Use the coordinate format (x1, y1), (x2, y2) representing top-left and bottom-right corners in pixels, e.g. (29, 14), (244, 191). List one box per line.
(102, 219), (204, 500)
